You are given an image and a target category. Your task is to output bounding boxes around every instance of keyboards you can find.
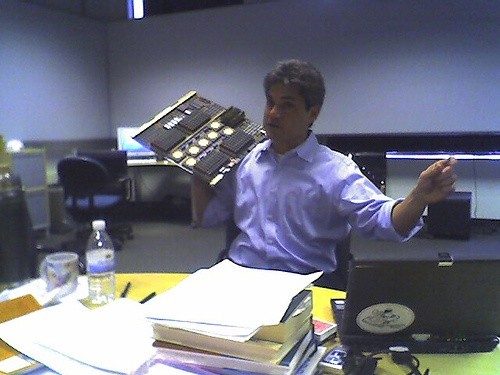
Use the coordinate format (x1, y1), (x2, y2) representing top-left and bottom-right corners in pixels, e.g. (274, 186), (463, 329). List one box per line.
(128, 155), (156, 159)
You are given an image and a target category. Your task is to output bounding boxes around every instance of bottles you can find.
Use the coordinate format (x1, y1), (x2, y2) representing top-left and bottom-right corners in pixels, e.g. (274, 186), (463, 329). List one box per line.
(86, 220), (116, 306)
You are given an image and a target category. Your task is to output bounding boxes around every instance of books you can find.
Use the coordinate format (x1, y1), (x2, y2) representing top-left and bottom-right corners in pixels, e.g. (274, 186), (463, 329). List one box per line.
(150, 289), (339, 375)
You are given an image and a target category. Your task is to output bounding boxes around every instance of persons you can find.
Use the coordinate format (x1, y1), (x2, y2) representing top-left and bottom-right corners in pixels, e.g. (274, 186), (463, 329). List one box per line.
(189, 58), (457, 292)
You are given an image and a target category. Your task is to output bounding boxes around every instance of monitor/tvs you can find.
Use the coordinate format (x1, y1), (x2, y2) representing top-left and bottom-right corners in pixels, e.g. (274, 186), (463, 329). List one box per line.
(116, 127), (157, 156)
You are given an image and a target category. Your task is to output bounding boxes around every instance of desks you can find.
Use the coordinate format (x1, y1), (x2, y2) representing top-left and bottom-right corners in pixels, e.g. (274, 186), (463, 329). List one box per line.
(127, 157), (213, 229)
(0, 273), (500, 375)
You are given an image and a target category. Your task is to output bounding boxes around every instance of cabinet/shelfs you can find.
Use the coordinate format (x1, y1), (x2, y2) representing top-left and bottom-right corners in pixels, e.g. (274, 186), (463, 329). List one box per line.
(13, 149), (51, 242)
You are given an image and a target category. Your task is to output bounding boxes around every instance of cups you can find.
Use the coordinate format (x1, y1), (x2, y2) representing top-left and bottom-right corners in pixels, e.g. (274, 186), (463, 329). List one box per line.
(43, 252), (78, 293)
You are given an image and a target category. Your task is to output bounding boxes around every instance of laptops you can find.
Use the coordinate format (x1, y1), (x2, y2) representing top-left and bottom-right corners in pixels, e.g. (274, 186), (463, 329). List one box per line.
(330, 259), (500, 353)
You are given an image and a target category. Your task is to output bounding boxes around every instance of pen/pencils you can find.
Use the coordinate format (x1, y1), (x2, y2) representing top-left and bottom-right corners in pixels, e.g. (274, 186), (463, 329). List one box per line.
(121, 281), (131, 298)
(139, 292), (156, 304)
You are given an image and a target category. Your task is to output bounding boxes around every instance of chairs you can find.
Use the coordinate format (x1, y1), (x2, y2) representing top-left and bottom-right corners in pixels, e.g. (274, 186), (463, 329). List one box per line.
(218, 215), (353, 291)
(56, 149), (135, 251)
